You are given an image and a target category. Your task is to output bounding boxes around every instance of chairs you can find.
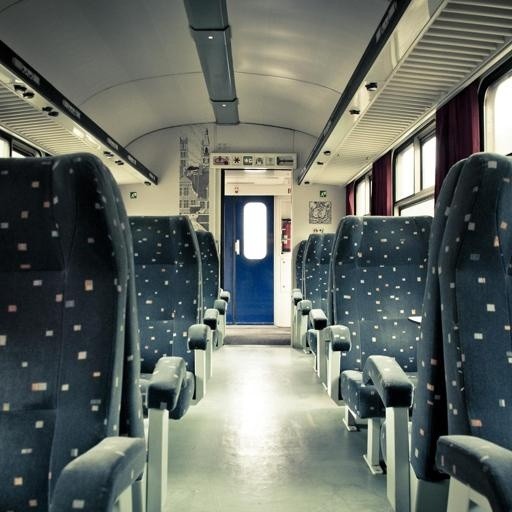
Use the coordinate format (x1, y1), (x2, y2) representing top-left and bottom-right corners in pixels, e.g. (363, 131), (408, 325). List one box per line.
(292, 240), (306, 349)
(308, 216), (345, 390)
(105, 165), (183, 511)
(196, 230), (228, 349)
(434, 151), (512, 511)
(128, 215), (211, 421)
(0, 153), (146, 512)
(322, 216), (432, 476)
(362, 156), (469, 511)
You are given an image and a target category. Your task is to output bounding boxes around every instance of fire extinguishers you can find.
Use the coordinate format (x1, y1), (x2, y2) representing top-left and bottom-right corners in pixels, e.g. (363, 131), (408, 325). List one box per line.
(282, 218), (291, 252)
